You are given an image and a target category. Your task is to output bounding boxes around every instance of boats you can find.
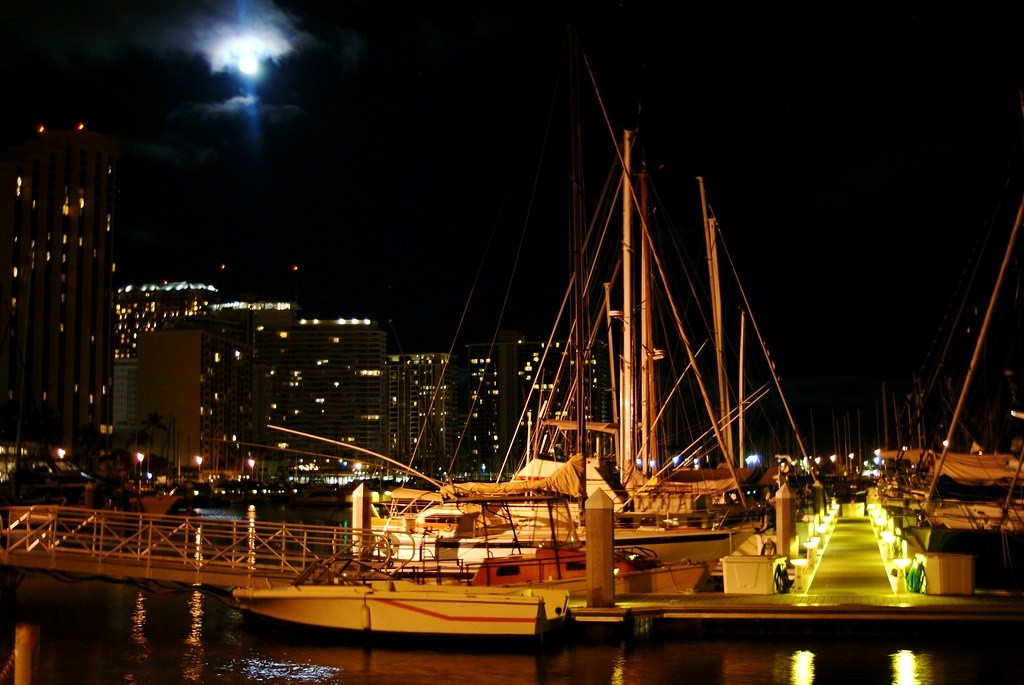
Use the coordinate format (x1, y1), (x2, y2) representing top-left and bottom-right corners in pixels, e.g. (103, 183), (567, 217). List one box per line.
(199, 529), (570, 645)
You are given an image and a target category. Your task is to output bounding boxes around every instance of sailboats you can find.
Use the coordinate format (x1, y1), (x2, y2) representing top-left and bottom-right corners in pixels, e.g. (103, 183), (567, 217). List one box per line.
(202, 13), (1024, 594)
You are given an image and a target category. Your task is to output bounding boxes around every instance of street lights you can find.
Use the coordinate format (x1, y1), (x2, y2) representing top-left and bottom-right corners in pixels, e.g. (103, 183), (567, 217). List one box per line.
(196, 456), (202, 482)
(249, 459), (255, 480)
(137, 453), (144, 496)
